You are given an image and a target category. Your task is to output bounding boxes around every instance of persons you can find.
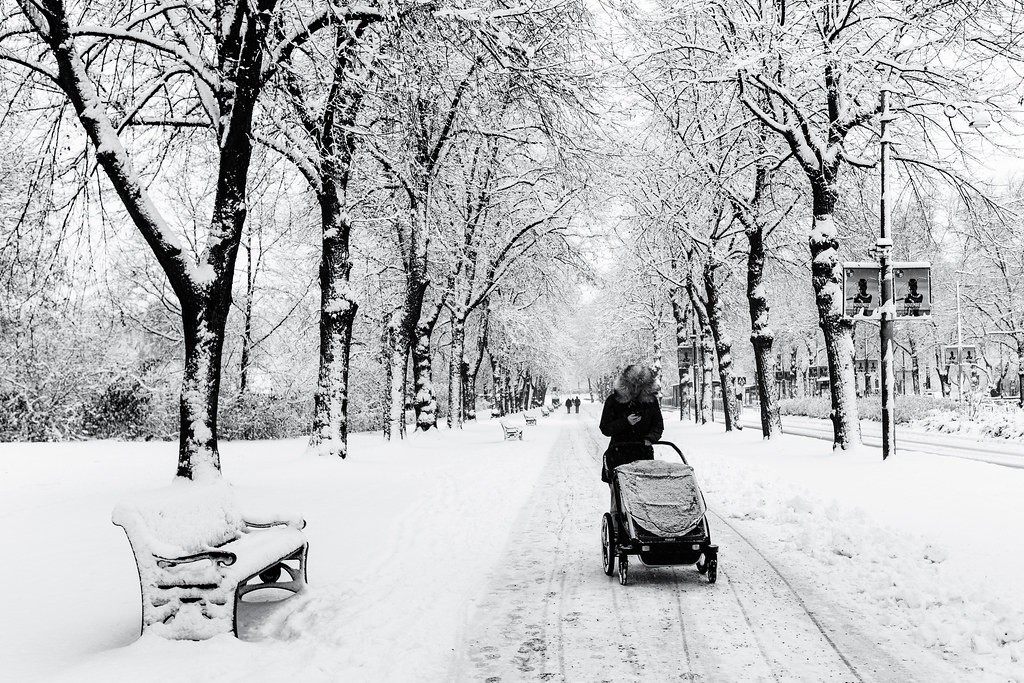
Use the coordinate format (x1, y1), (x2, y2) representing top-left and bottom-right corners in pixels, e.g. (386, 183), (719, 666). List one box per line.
(966, 350), (972, 359)
(859, 362), (863, 370)
(853, 279), (872, 316)
(904, 278), (923, 317)
(871, 363), (876, 369)
(565, 396), (581, 413)
(949, 351), (956, 360)
(598, 364), (664, 528)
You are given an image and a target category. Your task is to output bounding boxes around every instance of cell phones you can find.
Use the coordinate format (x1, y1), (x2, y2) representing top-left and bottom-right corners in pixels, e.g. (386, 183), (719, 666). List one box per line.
(635, 411), (643, 419)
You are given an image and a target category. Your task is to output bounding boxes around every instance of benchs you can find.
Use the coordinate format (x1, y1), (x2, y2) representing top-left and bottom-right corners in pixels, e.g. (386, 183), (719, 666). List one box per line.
(547, 406), (554, 412)
(524, 413), (537, 426)
(552, 403), (558, 409)
(540, 408), (549, 417)
(110, 480), (309, 642)
(499, 420), (524, 441)
(556, 402), (560, 407)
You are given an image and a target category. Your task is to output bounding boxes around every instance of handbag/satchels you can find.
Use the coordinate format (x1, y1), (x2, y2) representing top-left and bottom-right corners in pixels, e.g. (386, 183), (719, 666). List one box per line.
(601, 448), (616, 484)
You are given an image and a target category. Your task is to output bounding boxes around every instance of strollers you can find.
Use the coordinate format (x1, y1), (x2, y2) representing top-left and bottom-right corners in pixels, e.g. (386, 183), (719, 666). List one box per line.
(601, 440), (718, 584)
